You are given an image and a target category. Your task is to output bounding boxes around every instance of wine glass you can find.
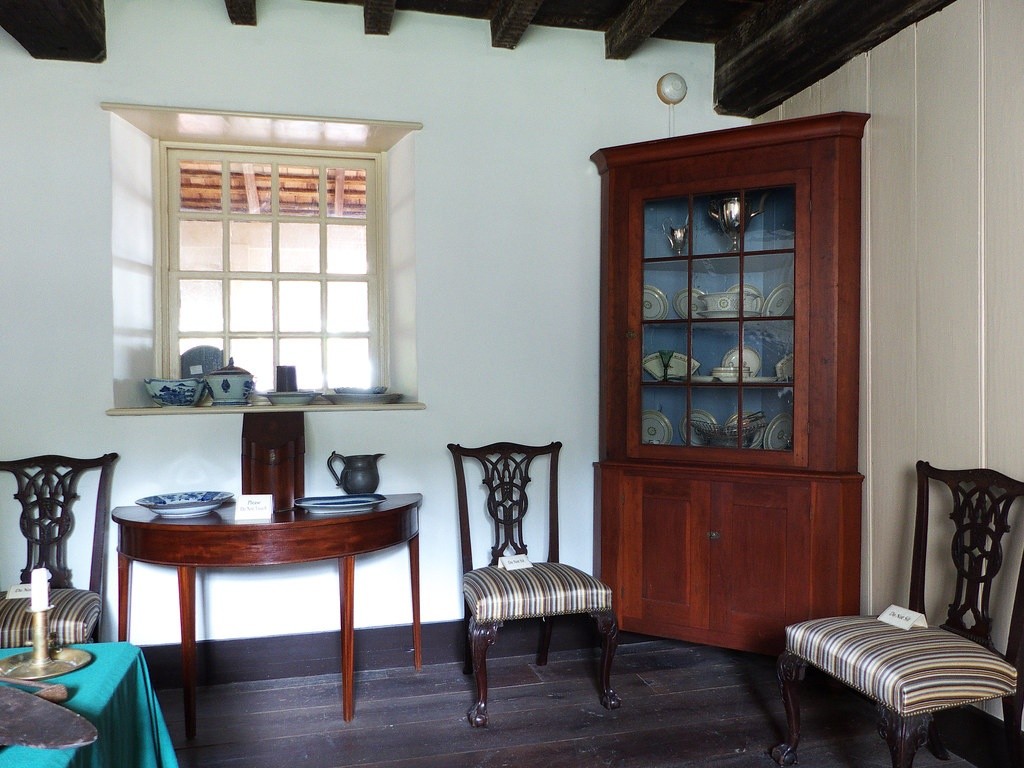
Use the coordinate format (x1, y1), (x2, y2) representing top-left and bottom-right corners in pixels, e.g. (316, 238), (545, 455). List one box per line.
(658, 350), (674, 381)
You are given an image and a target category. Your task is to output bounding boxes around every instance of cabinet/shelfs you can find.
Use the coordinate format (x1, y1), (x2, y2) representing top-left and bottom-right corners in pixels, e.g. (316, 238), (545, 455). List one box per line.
(585, 108), (872, 651)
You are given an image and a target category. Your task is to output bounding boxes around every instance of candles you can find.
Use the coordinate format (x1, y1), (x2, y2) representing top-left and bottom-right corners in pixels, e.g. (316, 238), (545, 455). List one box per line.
(30, 568), (50, 609)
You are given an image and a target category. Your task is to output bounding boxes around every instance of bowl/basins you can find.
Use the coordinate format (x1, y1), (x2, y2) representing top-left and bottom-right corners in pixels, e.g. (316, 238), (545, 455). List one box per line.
(202, 356), (257, 406)
(143, 378), (209, 409)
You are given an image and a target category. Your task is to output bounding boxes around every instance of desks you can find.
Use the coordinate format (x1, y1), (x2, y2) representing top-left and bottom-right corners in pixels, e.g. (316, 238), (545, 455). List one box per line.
(111, 493), (424, 741)
(0, 642), (182, 768)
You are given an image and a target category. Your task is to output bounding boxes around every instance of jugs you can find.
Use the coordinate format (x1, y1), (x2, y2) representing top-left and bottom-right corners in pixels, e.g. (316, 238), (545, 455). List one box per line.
(328, 451), (385, 493)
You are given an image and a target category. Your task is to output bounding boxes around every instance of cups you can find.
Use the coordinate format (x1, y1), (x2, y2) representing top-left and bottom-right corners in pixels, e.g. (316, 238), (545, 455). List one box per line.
(699, 292), (740, 310)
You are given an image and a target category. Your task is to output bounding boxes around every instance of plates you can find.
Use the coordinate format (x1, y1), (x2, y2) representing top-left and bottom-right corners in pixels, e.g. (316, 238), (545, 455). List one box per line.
(679, 409), (717, 446)
(695, 310), (762, 317)
(726, 284), (762, 312)
(775, 353), (793, 382)
(642, 409), (673, 445)
(295, 494), (387, 513)
(673, 289), (705, 318)
(761, 284), (794, 316)
(135, 491), (235, 518)
(724, 412), (766, 449)
(764, 412), (793, 450)
(642, 285), (669, 320)
(679, 375), (714, 382)
(719, 346), (776, 382)
(255, 393), (323, 405)
(642, 352), (701, 381)
(321, 386), (404, 405)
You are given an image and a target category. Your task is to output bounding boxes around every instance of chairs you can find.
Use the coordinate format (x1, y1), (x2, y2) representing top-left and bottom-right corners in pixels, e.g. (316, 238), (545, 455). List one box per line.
(771, 460), (1024, 768)
(0, 453), (120, 648)
(447, 440), (623, 729)
